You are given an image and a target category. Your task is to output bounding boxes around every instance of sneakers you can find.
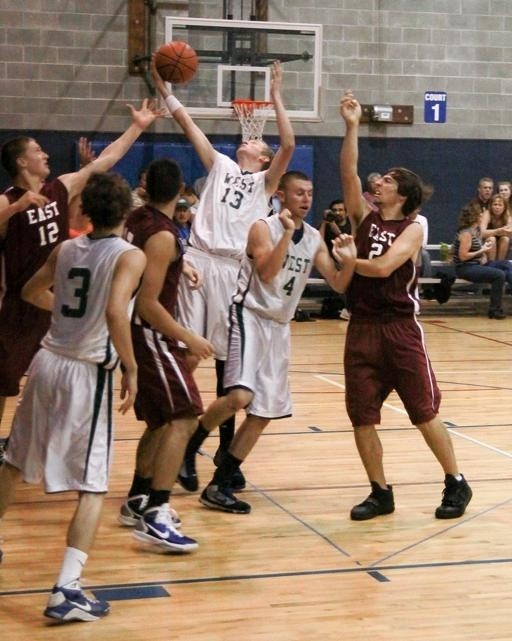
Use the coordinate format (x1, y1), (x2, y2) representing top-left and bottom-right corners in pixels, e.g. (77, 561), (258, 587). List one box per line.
(116, 494), (183, 526)
(350, 480), (394, 520)
(198, 478), (251, 512)
(435, 474), (474, 519)
(176, 454), (199, 491)
(488, 308), (505, 319)
(43, 584), (111, 622)
(130, 505), (199, 554)
(213, 448), (246, 488)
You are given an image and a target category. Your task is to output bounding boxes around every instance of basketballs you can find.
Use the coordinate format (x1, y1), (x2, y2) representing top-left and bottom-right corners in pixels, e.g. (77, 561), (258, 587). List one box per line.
(155, 39), (199, 85)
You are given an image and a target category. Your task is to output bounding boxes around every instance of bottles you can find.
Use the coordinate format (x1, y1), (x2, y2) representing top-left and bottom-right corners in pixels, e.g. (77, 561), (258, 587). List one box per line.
(440, 244), (448, 262)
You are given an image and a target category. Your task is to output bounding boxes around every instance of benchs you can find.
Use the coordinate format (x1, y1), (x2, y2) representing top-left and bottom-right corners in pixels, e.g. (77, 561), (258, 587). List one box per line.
(296, 243), (508, 316)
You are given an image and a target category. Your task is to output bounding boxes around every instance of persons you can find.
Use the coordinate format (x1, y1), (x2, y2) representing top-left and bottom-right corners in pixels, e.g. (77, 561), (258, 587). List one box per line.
(119, 156), (221, 556)
(173, 170), (361, 516)
(2, 169), (149, 626)
(67, 167), (200, 316)
(451, 179), (512, 320)
(146, 50), (300, 494)
(318, 173), (435, 320)
(336, 90), (475, 522)
(0, 96), (172, 465)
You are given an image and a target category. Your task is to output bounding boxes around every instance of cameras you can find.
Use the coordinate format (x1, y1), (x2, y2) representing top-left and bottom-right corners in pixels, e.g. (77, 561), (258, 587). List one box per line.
(327, 212), (343, 223)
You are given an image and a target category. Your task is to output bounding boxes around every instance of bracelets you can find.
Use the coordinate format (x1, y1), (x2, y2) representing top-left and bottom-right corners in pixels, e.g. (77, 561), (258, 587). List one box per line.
(164, 93), (185, 115)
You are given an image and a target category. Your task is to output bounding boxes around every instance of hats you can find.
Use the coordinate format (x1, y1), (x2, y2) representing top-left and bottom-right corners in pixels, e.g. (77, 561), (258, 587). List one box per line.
(174, 198), (190, 209)
